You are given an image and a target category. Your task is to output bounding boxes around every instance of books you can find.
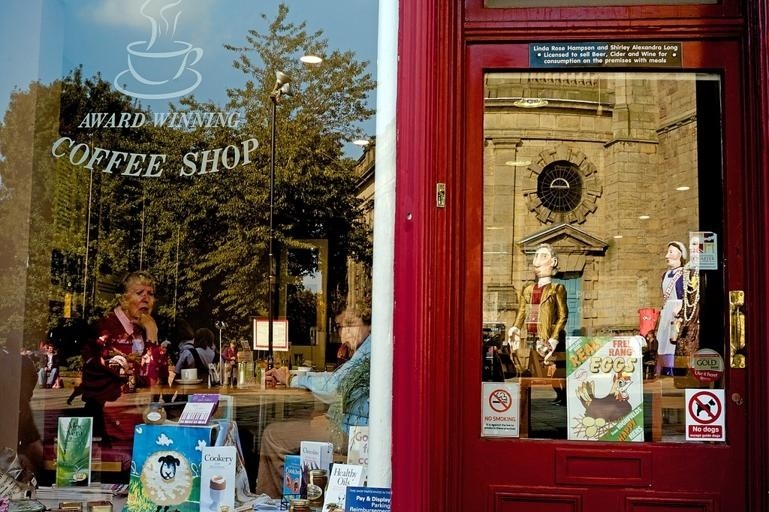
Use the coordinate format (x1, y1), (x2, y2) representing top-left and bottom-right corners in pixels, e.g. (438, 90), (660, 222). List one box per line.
(282, 426), (370, 512)
(199, 446), (237, 512)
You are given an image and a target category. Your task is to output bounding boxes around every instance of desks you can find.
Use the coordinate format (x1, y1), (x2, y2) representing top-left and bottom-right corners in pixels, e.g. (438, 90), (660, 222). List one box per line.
(136, 386), (327, 424)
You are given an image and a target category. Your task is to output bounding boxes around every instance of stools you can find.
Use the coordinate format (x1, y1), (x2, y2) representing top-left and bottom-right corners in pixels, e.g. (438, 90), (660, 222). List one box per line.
(43, 441), (132, 472)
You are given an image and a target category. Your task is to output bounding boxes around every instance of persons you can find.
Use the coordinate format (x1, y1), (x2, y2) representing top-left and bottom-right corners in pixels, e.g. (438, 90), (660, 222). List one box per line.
(4, 271), (372, 502)
(655, 240), (694, 377)
(506, 241), (568, 371)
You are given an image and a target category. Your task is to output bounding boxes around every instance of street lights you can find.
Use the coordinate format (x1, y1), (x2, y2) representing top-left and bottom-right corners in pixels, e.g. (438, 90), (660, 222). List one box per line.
(214, 320), (228, 386)
(82, 132), (96, 322)
(266, 69), (296, 380)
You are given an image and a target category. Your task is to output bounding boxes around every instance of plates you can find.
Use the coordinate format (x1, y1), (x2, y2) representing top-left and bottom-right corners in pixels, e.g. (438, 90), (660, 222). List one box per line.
(175, 379), (203, 385)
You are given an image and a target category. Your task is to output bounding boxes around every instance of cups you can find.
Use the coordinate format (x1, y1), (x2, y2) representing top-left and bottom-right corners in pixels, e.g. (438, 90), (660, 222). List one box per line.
(180, 368), (198, 380)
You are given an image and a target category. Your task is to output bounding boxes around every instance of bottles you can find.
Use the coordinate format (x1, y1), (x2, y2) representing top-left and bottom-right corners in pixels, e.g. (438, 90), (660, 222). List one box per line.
(141, 401), (167, 424)
(238, 362), (247, 388)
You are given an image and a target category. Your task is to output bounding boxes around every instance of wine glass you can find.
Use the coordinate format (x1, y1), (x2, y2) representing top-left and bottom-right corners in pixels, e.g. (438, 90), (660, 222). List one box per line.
(208, 488), (227, 511)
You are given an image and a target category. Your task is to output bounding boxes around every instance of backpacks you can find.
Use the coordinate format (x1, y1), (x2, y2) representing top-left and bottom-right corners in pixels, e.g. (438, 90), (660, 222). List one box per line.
(187, 347), (221, 382)
